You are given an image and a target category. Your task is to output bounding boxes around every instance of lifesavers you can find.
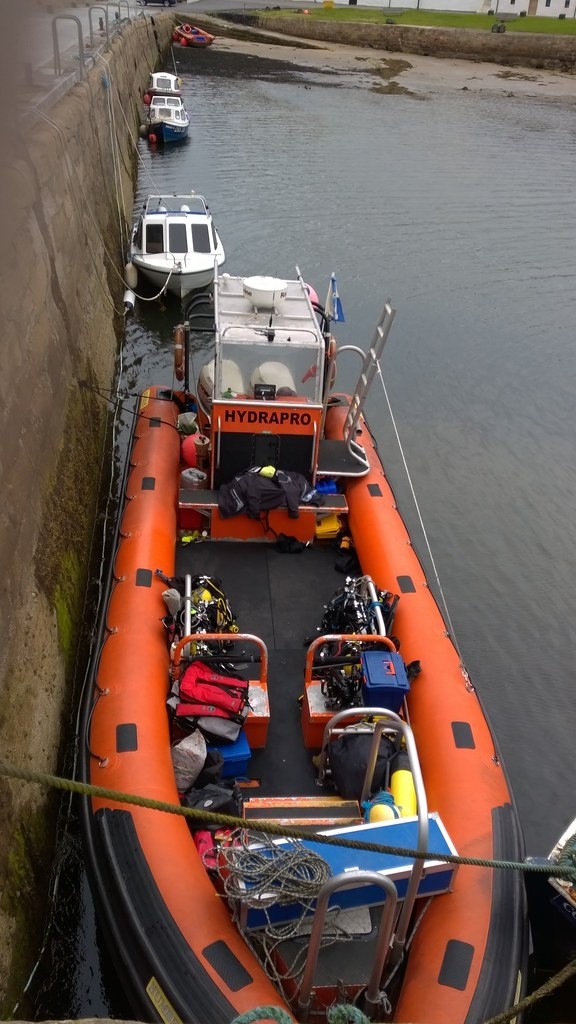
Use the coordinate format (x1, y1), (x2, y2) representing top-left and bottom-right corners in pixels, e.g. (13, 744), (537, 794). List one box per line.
(173, 324), (186, 380)
(329, 333), (336, 388)
(182, 24), (192, 33)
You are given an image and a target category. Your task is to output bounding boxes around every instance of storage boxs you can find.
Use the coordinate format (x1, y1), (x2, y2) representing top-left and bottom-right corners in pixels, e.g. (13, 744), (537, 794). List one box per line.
(205, 729), (253, 779)
(360, 650), (410, 713)
(231, 812), (461, 931)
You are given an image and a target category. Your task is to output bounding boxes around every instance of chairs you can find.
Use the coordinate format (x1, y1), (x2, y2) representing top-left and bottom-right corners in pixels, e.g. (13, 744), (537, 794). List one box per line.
(303, 634), (404, 754)
(171, 633), (272, 749)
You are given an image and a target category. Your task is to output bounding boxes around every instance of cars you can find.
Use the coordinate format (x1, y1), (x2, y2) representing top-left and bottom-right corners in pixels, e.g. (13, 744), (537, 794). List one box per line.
(136, 0), (177, 7)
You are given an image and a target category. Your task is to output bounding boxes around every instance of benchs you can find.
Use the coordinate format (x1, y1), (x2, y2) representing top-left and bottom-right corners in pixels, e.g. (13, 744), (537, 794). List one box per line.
(177, 487), (349, 544)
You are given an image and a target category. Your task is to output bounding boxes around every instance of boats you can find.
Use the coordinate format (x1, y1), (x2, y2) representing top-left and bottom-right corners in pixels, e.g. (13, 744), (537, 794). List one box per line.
(127, 186), (228, 299)
(525, 816), (575, 936)
(174, 23), (214, 48)
(79, 249), (539, 1024)
(144, 94), (191, 143)
(146, 71), (183, 97)
